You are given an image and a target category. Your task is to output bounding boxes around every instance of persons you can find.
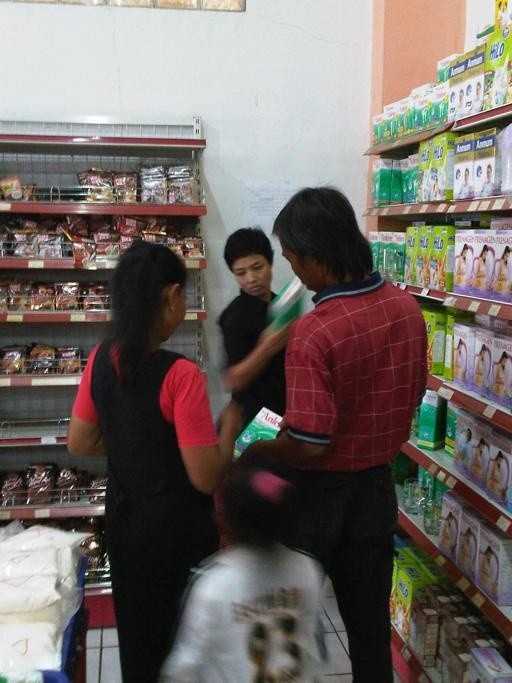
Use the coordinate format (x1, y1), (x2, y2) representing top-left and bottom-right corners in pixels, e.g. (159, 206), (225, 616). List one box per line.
(157, 455), (330, 682)
(215, 226), (296, 436)
(407, 606), (422, 651)
(394, 597), (406, 631)
(453, 83), (497, 201)
(439, 428), (508, 597)
(453, 239), (510, 398)
(234, 187), (430, 683)
(65, 237), (244, 682)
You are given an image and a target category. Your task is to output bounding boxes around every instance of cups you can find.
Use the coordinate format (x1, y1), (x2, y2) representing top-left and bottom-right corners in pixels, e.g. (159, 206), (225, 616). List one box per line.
(403, 478), (442, 535)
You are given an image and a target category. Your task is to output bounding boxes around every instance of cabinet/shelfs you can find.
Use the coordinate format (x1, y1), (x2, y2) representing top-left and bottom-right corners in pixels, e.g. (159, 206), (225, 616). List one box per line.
(0, 116), (208, 630)
(365, 104), (511, 683)
